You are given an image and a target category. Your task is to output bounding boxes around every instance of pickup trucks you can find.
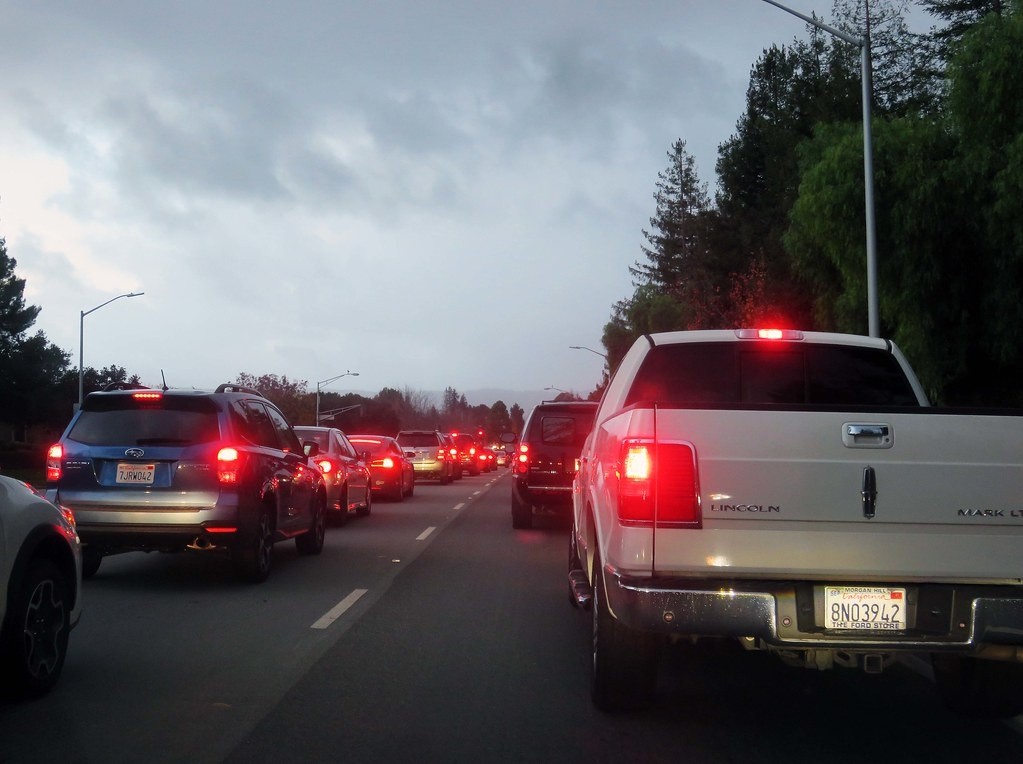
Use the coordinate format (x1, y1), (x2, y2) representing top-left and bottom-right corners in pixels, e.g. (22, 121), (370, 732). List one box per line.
(564, 331), (1022, 705)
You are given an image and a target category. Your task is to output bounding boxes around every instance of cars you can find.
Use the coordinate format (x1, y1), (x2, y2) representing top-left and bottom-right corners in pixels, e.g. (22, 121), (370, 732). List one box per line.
(0, 476), (88, 700)
(346, 435), (417, 501)
(444, 435), (512, 476)
(289, 425), (372, 523)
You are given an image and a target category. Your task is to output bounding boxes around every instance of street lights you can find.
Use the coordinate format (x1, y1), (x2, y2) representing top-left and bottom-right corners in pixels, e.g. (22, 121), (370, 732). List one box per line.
(77, 292), (148, 410)
(316, 370), (360, 426)
(570, 346), (611, 384)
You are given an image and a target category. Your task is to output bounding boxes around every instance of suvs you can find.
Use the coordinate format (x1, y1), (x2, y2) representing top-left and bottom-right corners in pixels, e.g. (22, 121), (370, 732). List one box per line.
(46, 378), (328, 582)
(500, 403), (596, 528)
(396, 430), (454, 484)
